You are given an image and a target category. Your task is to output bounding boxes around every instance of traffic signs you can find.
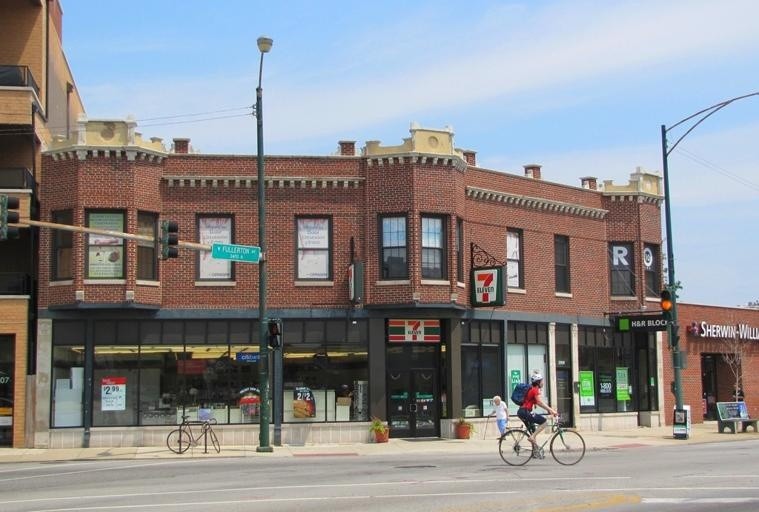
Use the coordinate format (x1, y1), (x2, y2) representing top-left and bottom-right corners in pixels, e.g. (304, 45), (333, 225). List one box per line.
(211, 243), (261, 264)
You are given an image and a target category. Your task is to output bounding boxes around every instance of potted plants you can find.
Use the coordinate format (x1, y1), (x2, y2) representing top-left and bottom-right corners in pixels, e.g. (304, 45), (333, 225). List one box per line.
(369, 415), (391, 443)
(454, 414), (478, 438)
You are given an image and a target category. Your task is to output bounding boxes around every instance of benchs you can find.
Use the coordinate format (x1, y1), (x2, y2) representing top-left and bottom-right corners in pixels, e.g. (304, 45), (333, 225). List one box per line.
(716, 401), (758, 434)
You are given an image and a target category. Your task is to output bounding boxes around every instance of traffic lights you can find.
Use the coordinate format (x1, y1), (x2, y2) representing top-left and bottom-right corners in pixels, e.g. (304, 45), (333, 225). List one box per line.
(268, 322), (282, 348)
(0, 193), (20, 241)
(156, 219), (180, 259)
(660, 290), (672, 322)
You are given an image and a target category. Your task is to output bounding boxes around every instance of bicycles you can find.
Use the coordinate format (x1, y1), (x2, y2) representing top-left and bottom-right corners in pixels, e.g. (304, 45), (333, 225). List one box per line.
(497, 410), (585, 466)
(166, 415), (221, 454)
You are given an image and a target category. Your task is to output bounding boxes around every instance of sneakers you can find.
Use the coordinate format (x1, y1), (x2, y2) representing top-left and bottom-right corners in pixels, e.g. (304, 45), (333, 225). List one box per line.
(527, 435), (538, 446)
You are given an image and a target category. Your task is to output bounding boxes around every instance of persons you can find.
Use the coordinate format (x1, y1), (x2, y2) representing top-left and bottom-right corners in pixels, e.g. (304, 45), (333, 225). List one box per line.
(732, 383), (744, 401)
(517, 374), (558, 458)
(488, 395), (509, 440)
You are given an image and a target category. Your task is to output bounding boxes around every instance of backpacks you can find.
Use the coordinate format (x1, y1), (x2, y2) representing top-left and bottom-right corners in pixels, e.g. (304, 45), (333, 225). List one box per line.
(511, 383), (532, 406)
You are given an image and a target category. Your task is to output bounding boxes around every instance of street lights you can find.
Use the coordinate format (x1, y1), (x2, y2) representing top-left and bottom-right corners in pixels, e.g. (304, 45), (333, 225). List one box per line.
(254, 33), (273, 452)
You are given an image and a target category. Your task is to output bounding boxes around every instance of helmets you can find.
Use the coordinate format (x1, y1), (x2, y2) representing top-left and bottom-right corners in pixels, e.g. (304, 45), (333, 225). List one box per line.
(531, 373), (543, 383)
